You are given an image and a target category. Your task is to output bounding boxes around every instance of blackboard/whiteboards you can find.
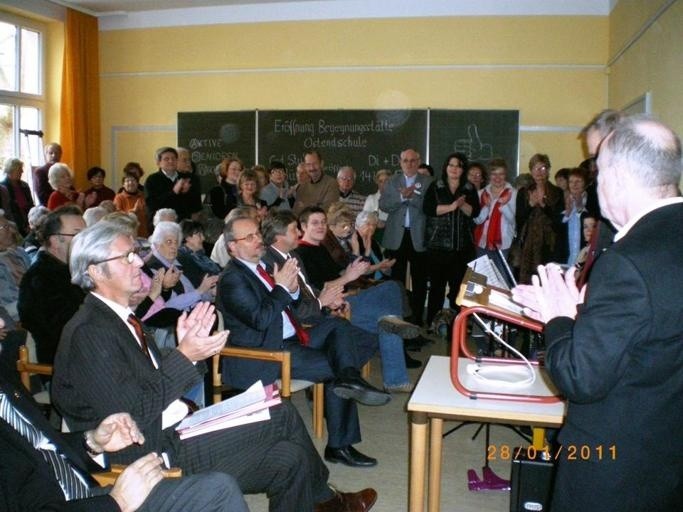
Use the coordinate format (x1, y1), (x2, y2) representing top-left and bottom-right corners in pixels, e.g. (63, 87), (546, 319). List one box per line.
(429, 111), (519, 182)
(177, 110), (256, 198)
(257, 108), (429, 197)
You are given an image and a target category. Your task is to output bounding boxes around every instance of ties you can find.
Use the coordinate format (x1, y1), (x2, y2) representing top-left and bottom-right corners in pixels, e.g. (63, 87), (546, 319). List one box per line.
(0, 394), (92, 501)
(124, 313), (200, 414)
(254, 262), (312, 348)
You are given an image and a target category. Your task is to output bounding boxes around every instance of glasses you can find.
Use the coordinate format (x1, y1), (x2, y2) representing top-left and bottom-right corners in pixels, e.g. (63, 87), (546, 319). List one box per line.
(229, 231), (261, 242)
(0, 223), (12, 232)
(86, 248), (137, 267)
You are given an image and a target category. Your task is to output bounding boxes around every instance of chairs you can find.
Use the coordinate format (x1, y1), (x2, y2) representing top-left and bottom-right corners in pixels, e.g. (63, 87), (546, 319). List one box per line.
(212, 331), (324, 439)
(16, 345), (54, 390)
(91, 464), (181, 488)
(13, 288), (376, 512)
(331, 291), (358, 321)
(362, 360), (369, 379)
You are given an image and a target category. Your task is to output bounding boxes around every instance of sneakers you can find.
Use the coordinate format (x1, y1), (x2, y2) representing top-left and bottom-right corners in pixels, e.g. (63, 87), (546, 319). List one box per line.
(382, 383), (416, 393)
(377, 315), (421, 342)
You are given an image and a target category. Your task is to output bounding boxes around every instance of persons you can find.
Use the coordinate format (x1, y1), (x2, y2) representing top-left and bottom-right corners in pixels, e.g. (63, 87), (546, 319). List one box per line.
(508, 112), (681, 509)
(0, 346), (251, 510)
(578, 106), (632, 158)
(47, 217), (379, 511)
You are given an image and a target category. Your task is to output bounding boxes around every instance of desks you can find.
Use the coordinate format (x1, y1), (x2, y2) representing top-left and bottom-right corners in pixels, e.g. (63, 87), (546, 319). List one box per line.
(405, 355), (570, 512)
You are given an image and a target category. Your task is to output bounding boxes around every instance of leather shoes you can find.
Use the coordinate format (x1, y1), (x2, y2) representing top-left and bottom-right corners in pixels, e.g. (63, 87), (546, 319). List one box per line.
(323, 443), (379, 468)
(404, 356), (424, 370)
(329, 371), (392, 408)
(405, 336), (437, 352)
(311, 482), (379, 512)
